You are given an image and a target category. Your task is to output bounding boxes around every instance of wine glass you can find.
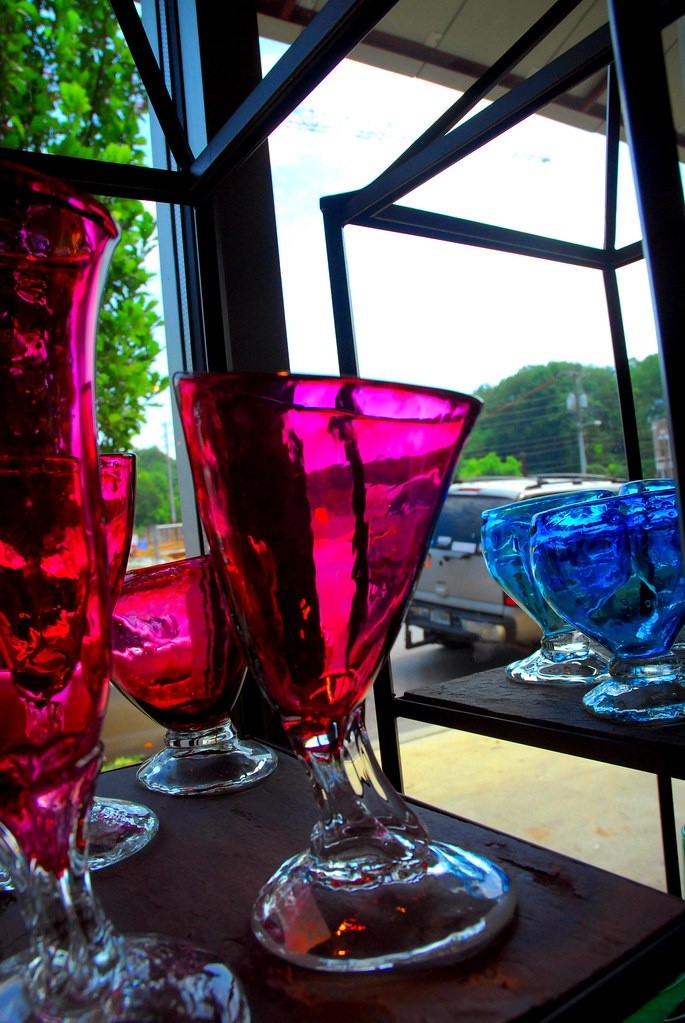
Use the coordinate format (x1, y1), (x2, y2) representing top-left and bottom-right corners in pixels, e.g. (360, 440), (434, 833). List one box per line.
(176, 371), (513, 972)
(0, 158), (250, 1022)
(481, 478), (685, 723)
(107, 554), (278, 795)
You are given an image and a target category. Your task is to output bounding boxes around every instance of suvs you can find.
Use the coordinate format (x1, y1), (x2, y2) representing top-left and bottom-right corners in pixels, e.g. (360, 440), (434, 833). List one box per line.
(404, 472), (629, 649)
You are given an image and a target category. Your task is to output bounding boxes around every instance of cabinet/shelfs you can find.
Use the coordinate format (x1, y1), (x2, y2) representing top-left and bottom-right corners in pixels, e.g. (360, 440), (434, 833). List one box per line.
(318, 0), (685, 899)
(0, 0), (685, 1023)
